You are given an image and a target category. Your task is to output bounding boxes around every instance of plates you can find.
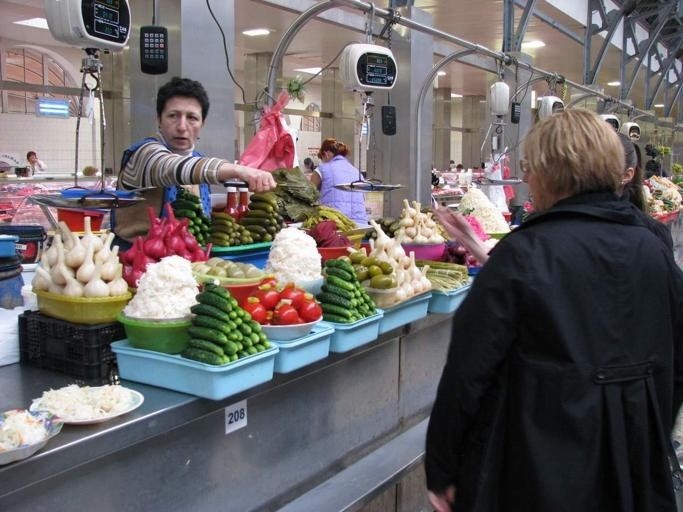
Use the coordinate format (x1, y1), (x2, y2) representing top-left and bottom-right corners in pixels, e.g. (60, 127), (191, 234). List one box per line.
(0, 385), (145, 466)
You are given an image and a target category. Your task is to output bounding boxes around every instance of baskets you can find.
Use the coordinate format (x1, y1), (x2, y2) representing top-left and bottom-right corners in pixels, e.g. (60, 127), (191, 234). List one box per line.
(18, 309), (125, 385)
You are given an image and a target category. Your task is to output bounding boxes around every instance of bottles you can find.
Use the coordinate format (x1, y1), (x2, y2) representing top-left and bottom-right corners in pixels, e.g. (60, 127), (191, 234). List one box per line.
(105, 167), (112, 191)
(459, 168), (473, 187)
(225, 187), (248, 221)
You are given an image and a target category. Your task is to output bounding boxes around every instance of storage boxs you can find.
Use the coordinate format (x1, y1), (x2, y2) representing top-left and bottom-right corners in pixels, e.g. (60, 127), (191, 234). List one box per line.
(427, 285), (472, 314)
(112, 324), (278, 402)
(315, 308), (383, 354)
(376, 286), (433, 337)
(268, 325), (335, 374)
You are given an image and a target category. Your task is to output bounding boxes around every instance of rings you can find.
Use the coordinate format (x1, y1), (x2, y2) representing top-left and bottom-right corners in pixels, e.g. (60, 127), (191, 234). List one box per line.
(262, 183), (269, 186)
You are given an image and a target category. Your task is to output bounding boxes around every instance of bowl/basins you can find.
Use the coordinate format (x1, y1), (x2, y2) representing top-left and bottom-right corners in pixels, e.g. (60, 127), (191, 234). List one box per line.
(191, 271), (264, 286)
(261, 316), (323, 340)
(317, 247), (352, 263)
(59, 207), (102, 229)
(403, 243), (445, 261)
(115, 312), (192, 355)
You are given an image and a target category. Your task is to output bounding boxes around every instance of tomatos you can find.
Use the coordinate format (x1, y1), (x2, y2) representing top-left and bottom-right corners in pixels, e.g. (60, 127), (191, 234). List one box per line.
(240, 276), (323, 324)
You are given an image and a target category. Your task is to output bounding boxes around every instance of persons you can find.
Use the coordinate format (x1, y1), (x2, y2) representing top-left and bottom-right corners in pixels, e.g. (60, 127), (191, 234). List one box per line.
(421, 105), (683, 511)
(25, 151), (48, 172)
(82, 166), (96, 176)
(112, 76), (278, 252)
(303, 157), (315, 172)
(310, 137), (369, 225)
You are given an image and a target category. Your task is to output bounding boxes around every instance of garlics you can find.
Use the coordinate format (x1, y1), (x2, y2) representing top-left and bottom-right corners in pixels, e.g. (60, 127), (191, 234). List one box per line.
(370, 198), (444, 304)
(30, 204), (213, 299)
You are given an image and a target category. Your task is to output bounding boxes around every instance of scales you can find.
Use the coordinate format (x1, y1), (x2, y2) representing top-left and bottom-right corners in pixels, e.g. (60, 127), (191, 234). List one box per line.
(29, 0), (145, 210)
(538, 96), (565, 119)
(620, 122), (641, 141)
(332, 44), (406, 191)
(487, 82), (522, 185)
(599, 114), (620, 133)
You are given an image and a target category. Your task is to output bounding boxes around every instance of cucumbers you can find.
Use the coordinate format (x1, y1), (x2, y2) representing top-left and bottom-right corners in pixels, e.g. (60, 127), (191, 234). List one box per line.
(173, 186), (284, 247)
(182, 282), (272, 366)
(317, 258), (378, 324)
